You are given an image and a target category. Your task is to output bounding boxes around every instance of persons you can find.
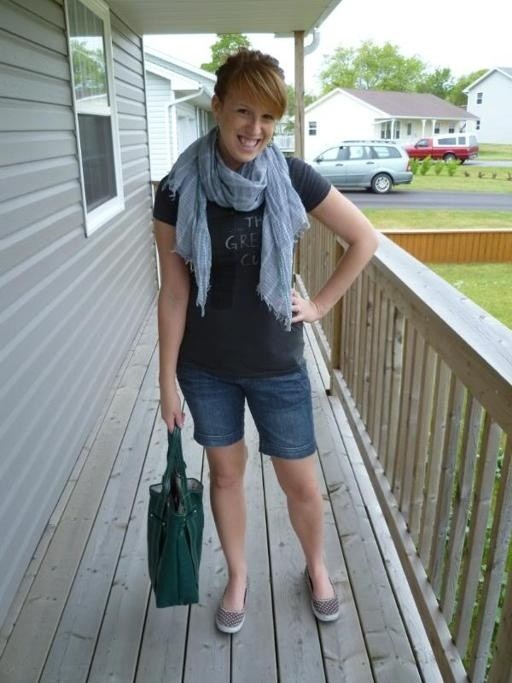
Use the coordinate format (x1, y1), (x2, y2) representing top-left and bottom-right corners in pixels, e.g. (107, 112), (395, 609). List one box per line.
(152, 46), (381, 636)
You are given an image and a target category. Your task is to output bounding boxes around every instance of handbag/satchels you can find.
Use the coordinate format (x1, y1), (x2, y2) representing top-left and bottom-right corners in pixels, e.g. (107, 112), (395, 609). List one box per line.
(148, 430), (205, 607)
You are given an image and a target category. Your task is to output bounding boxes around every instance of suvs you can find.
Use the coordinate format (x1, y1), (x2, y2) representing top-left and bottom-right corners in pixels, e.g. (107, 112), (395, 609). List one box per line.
(305, 138), (415, 195)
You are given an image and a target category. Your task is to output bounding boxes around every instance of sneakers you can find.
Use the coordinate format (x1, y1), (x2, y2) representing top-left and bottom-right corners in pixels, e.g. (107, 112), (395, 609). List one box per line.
(306, 563), (341, 622)
(217, 575), (248, 633)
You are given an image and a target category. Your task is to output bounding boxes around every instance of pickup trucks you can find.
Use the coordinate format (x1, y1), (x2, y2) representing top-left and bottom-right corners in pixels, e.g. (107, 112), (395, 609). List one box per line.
(400, 132), (480, 167)
